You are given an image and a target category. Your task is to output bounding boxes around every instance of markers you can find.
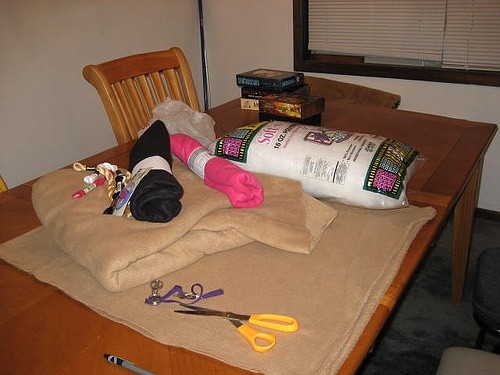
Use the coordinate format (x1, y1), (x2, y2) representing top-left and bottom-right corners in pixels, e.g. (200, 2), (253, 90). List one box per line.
(71, 177), (106, 199)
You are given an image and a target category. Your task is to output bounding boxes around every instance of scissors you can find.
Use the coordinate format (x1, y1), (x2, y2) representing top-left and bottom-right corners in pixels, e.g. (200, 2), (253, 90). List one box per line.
(174, 302), (298, 352)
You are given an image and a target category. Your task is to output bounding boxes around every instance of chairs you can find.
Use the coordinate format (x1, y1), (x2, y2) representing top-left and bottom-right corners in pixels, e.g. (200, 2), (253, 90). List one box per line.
(82, 46), (203, 145)
(471, 247), (500, 350)
(303, 75), (401, 109)
(435, 347), (500, 375)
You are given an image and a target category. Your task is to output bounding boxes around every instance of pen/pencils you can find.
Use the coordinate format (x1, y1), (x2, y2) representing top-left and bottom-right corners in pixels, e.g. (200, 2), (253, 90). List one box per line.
(102, 354), (155, 375)
